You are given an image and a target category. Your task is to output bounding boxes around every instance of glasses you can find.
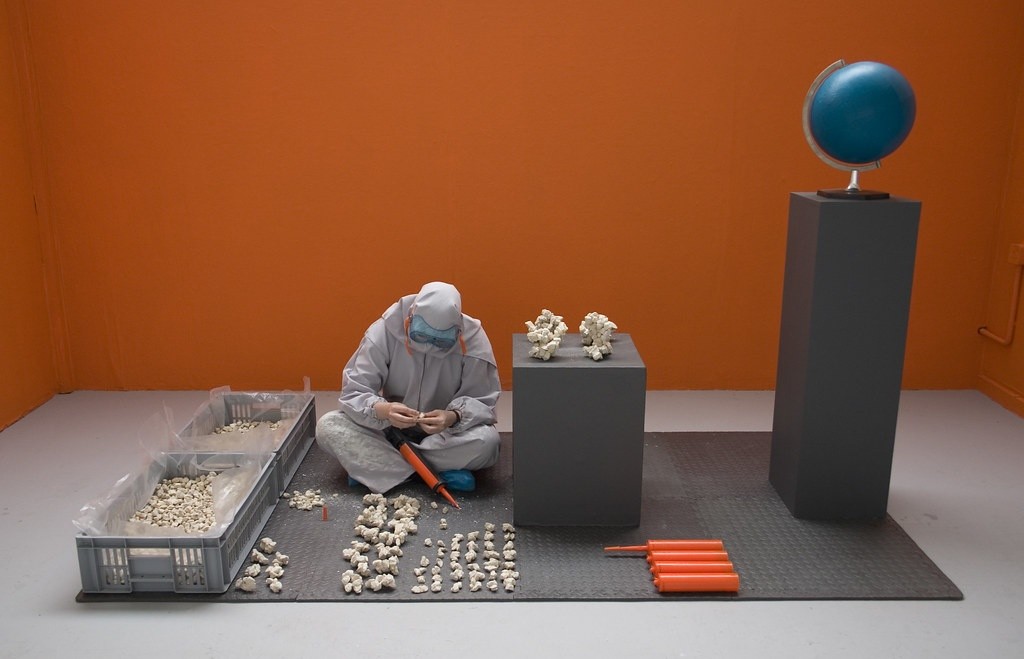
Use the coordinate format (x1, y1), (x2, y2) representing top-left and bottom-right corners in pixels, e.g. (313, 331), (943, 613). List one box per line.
(405, 314), (459, 349)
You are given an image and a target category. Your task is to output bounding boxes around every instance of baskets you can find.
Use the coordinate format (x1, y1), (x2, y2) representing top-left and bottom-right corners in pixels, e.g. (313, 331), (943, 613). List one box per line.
(171, 391), (316, 496)
(75, 453), (280, 594)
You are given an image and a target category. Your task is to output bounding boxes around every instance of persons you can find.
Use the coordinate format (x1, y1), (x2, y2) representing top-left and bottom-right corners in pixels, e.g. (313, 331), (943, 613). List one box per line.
(316, 282), (502, 495)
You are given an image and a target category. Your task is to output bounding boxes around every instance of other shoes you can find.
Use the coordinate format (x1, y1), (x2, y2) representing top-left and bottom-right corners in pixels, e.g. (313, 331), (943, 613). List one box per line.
(436, 470), (475, 492)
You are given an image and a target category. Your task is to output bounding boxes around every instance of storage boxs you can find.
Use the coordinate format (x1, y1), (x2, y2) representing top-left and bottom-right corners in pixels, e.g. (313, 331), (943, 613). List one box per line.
(74, 450), (280, 596)
(170, 391), (318, 494)
